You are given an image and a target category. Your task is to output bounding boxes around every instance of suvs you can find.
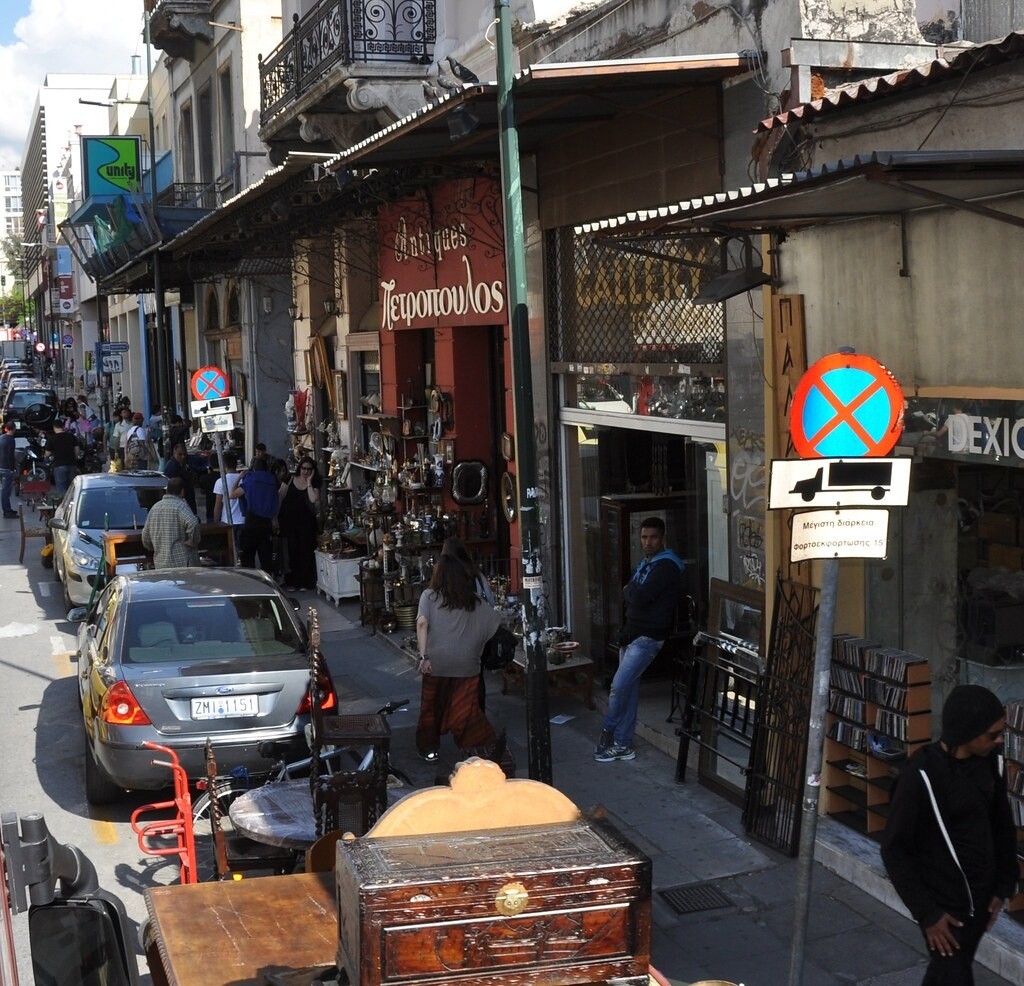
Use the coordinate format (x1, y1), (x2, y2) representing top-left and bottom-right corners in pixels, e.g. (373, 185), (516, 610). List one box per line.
(1, 388), (59, 433)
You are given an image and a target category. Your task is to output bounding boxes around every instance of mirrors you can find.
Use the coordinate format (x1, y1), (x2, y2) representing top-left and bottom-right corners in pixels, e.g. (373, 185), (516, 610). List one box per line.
(697, 577), (765, 810)
(501, 472), (517, 523)
(452, 461), (490, 504)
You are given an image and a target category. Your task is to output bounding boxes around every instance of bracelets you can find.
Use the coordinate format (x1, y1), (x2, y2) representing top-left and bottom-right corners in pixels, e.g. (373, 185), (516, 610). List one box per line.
(420, 655), (429, 660)
(307, 486), (312, 488)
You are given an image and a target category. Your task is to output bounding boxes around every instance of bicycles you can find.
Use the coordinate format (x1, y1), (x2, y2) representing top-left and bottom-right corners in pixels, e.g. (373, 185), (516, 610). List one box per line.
(182, 698), (412, 881)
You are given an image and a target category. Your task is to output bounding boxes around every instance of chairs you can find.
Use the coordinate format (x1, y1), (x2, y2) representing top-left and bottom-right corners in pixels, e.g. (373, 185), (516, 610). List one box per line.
(237, 618), (274, 643)
(18, 501), (52, 564)
(314, 768), (380, 840)
(205, 740), (296, 881)
(305, 827), (343, 873)
(139, 622), (179, 646)
(308, 606), (393, 799)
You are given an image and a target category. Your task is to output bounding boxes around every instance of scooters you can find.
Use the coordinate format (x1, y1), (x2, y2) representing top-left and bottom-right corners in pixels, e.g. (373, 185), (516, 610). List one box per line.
(13, 432), (48, 483)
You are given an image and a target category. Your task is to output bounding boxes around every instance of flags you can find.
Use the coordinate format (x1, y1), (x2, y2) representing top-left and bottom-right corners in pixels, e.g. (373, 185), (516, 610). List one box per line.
(93, 195), (142, 253)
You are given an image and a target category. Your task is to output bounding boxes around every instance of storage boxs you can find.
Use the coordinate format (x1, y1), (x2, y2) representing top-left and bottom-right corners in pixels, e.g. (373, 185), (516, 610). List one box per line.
(335, 816), (652, 986)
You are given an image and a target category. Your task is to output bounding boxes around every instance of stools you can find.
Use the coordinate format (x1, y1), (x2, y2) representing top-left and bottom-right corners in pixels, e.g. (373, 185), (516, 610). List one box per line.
(36, 506), (58, 521)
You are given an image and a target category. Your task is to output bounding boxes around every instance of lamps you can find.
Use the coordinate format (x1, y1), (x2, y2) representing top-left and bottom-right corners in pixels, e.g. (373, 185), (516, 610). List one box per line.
(323, 297), (341, 317)
(362, 514), (388, 559)
(287, 302), (303, 321)
(692, 266), (778, 304)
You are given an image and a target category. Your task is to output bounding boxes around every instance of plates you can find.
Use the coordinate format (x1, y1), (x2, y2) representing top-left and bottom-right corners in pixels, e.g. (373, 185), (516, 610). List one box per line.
(554, 641), (580, 653)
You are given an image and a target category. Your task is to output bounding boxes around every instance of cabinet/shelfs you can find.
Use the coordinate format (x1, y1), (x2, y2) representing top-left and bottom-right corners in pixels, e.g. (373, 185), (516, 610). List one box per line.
(826, 646), (932, 843)
(313, 550), (367, 607)
(397, 392), (428, 503)
(350, 413), (404, 514)
(1002, 698), (1024, 926)
(286, 420), (316, 473)
(600, 490), (694, 697)
(383, 534), (499, 612)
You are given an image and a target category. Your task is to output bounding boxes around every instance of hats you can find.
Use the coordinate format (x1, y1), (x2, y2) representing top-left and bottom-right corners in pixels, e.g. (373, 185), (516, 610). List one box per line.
(941, 685), (1007, 744)
(134, 413), (142, 419)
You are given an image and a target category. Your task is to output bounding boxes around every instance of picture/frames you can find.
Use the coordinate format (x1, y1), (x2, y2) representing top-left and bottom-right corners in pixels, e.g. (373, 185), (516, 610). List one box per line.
(442, 439), (456, 468)
(501, 432), (514, 461)
(330, 369), (346, 420)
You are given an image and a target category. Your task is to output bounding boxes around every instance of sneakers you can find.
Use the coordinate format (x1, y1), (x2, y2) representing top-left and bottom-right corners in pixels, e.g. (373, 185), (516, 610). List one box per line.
(595, 741), (636, 761)
(594, 727), (614, 754)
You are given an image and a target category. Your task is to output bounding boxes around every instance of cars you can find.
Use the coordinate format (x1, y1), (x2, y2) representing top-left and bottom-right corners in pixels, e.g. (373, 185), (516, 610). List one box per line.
(47, 470), (172, 613)
(2, 358), (40, 390)
(68, 567), (341, 804)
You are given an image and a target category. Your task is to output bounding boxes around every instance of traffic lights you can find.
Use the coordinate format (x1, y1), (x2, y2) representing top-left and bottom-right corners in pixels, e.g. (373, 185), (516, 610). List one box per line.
(26, 895), (138, 986)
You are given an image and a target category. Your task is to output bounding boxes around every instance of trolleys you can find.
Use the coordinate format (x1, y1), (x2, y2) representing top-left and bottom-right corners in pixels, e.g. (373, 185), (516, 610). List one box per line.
(134, 741), (204, 885)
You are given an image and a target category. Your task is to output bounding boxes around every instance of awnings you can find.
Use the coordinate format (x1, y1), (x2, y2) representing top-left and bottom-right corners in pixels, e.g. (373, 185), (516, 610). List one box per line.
(102, 52), (767, 297)
(572, 148), (1023, 278)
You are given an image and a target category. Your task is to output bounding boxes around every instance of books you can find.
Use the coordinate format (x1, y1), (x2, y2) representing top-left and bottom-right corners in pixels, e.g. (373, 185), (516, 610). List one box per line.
(828, 633), (929, 780)
(1003, 699), (1024, 827)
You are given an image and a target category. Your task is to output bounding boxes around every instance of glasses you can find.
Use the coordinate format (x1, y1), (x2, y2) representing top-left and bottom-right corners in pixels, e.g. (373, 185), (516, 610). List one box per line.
(301, 466), (312, 471)
(178, 453), (186, 456)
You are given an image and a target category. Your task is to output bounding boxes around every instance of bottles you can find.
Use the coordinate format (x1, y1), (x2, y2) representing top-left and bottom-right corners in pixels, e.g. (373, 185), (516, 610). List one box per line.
(432, 454), (444, 487)
(424, 464), (434, 487)
(394, 511), (458, 547)
(398, 470), (411, 486)
(284, 390), (298, 430)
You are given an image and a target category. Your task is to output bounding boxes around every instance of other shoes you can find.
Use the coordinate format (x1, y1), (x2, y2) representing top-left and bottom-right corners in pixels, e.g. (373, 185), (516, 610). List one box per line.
(287, 585), (295, 591)
(300, 585), (307, 591)
(4, 513), (19, 518)
(13, 511), (17, 514)
(417, 749), (439, 761)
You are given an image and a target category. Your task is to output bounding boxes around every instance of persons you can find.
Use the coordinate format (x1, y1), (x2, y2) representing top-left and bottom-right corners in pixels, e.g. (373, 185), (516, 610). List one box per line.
(164, 444), (197, 514)
(45, 358), (74, 387)
(58, 395), (192, 470)
(255, 443), (289, 570)
(141, 477), (201, 569)
(0, 422), (20, 519)
(442, 537), (495, 711)
(272, 457), (322, 592)
(213, 454), (246, 553)
(44, 419), (82, 492)
(200, 453), (222, 519)
(229, 456), (279, 575)
(416, 553), (497, 762)
(880, 685), (1019, 986)
(594, 518), (685, 762)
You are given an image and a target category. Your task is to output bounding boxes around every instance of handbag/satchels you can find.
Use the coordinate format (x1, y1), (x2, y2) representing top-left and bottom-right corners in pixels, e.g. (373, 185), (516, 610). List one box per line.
(614, 628), (643, 646)
(108, 457), (122, 472)
(483, 625), (519, 670)
(126, 426), (145, 461)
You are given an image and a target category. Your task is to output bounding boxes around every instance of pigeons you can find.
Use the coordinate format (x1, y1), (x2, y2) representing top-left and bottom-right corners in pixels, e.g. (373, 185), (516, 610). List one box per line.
(420, 80), (446, 103)
(435, 61), (461, 89)
(444, 56), (479, 84)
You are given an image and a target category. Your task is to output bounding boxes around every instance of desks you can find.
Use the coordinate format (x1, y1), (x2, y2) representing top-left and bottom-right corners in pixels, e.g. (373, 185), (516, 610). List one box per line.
(143, 871), (668, 986)
(228, 775), (431, 875)
(103, 519), (235, 587)
(502, 641), (596, 711)
